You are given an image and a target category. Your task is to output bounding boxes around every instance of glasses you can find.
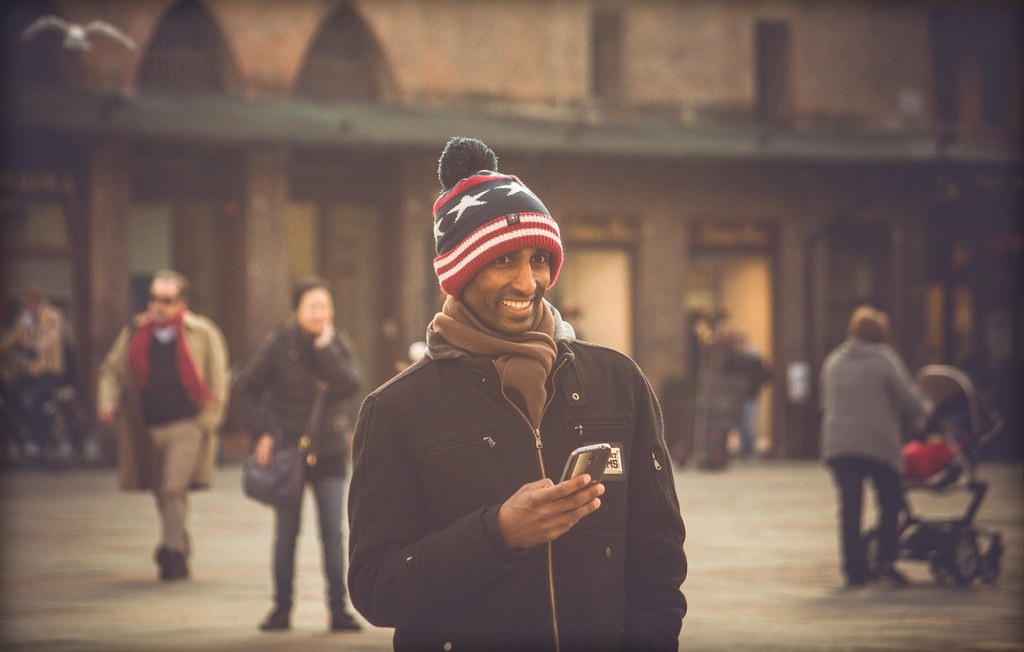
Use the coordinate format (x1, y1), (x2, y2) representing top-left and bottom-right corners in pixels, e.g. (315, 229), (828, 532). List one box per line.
(147, 292), (184, 304)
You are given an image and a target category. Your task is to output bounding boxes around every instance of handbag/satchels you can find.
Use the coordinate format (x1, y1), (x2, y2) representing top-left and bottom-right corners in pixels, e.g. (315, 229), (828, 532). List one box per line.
(241, 439), (319, 509)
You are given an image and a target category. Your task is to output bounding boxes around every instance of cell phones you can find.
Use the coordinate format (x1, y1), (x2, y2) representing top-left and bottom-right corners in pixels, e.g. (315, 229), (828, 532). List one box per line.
(559, 443), (611, 485)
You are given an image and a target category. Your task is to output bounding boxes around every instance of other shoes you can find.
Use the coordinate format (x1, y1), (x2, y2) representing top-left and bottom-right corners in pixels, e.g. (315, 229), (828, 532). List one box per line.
(157, 547), (189, 583)
(877, 562), (908, 591)
(846, 575), (872, 590)
(258, 600), (292, 630)
(327, 603), (362, 631)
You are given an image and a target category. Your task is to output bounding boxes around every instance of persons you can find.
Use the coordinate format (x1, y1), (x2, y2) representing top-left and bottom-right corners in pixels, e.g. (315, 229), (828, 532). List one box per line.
(346, 136), (688, 652)
(230, 276), (363, 633)
(899, 434), (960, 477)
(0, 299), (100, 467)
(817, 305), (935, 588)
(97, 268), (231, 581)
(552, 305), (772, 471)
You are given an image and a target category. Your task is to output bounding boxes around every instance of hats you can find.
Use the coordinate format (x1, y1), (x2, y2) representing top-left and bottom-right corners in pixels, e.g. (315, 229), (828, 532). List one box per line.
(433, 136), (565, 298)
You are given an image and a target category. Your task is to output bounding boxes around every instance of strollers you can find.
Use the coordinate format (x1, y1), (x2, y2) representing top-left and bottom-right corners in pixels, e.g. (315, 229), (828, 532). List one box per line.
(851, 365), (1003, 589)
